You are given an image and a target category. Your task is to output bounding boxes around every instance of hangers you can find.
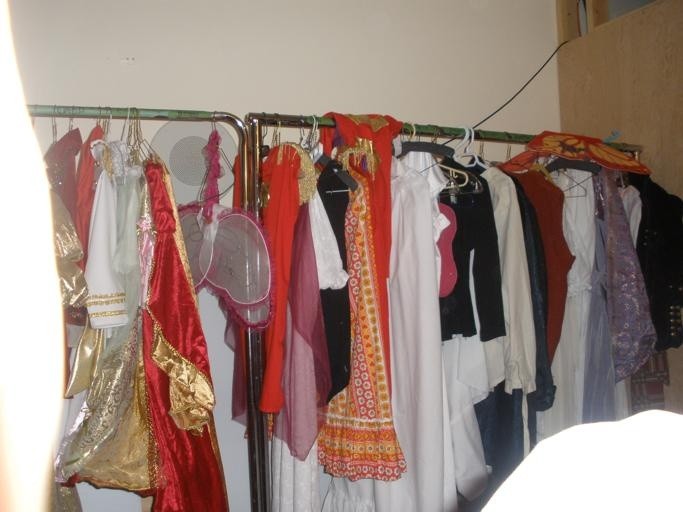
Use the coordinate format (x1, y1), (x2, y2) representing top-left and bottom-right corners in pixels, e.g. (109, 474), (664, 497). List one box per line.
(199, 110), (234, 202)
(260, 112), (605, 209)
(49, 104), (162, 172)
